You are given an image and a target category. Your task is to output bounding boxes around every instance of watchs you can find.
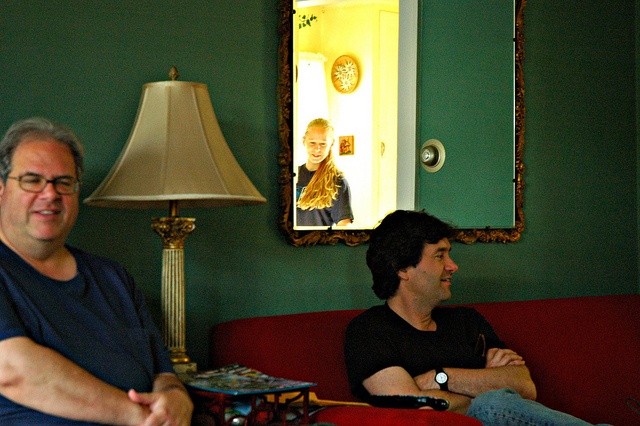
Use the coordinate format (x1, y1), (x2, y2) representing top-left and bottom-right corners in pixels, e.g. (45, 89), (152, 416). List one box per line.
(434, 366), (449, 390)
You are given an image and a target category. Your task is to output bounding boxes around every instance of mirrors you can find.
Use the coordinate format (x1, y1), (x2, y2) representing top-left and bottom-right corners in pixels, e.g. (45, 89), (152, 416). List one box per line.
(277, 0), (525, 245)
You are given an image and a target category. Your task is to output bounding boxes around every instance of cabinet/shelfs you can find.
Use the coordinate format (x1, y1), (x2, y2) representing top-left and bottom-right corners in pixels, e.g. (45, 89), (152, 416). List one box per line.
(182, 362), (318, 425)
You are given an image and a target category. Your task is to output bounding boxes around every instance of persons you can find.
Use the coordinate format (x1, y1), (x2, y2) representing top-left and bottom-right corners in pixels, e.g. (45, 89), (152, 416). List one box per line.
(345, 210), (595, 426)
(296, 117), (353, 226)
(0, 116), (194, 425)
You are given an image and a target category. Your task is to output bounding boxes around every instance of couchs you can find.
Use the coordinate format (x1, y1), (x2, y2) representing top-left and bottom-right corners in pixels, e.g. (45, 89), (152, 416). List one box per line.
(210, 296), (640, 424)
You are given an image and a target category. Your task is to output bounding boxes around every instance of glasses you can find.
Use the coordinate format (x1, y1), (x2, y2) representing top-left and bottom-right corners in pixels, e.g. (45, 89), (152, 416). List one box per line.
(5, 172), (80, 195)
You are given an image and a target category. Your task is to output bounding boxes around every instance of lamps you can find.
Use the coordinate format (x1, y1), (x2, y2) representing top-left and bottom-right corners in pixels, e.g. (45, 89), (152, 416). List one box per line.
(80, 67), (267, 372)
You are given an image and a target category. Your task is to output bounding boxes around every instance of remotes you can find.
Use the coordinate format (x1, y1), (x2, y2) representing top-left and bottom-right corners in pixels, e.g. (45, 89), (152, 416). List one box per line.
(369, 393), (449, 410)
